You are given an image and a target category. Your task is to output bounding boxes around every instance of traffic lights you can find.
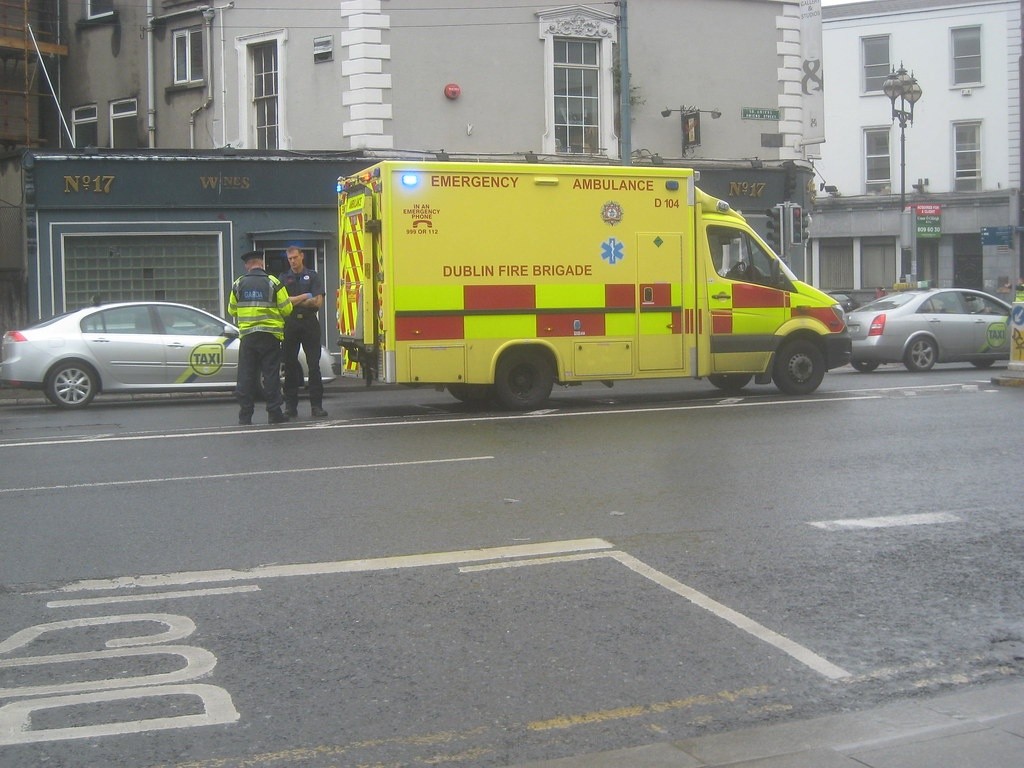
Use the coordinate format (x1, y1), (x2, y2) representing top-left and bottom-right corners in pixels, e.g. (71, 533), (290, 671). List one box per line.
(801, 208), (810, 243)
(789, 204), (803, 245)
(766, 204), (784, 255)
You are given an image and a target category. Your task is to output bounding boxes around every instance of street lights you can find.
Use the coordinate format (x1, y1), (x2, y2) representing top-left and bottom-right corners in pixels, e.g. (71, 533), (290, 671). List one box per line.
(882, 59), (922, 285)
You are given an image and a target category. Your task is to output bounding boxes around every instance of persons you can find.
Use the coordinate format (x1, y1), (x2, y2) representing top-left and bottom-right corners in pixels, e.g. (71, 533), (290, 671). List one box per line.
(228, 246), (328, 424)
(162, 310), (211, 336)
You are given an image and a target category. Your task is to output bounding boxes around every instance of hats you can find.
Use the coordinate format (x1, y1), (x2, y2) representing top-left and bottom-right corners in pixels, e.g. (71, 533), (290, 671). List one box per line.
(240, 251), (264, 261)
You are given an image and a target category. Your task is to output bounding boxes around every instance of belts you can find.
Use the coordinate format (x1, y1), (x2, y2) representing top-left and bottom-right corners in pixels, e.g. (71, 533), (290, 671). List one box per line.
(290, 312), (317, 319)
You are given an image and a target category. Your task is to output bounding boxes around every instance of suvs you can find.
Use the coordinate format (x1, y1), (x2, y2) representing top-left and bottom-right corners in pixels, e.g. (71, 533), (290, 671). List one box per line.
(822, 288), (856, 313)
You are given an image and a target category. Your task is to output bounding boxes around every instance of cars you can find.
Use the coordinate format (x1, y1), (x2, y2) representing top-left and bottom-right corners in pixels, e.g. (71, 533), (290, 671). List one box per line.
(0, 300), (338, 412)
(843, 287), (1013, 372)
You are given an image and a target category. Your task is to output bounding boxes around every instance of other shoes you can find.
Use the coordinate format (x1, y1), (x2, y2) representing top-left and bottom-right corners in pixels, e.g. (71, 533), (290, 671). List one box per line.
(312, 405), (328, 417)
(238, 416), (252, 424)
(283, 405), (298, 416)
(267, 412), (290, 424)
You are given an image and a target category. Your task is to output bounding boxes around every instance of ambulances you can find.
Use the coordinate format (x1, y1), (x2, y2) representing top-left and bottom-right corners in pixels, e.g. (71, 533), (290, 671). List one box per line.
(335, 160), (853, 412)
